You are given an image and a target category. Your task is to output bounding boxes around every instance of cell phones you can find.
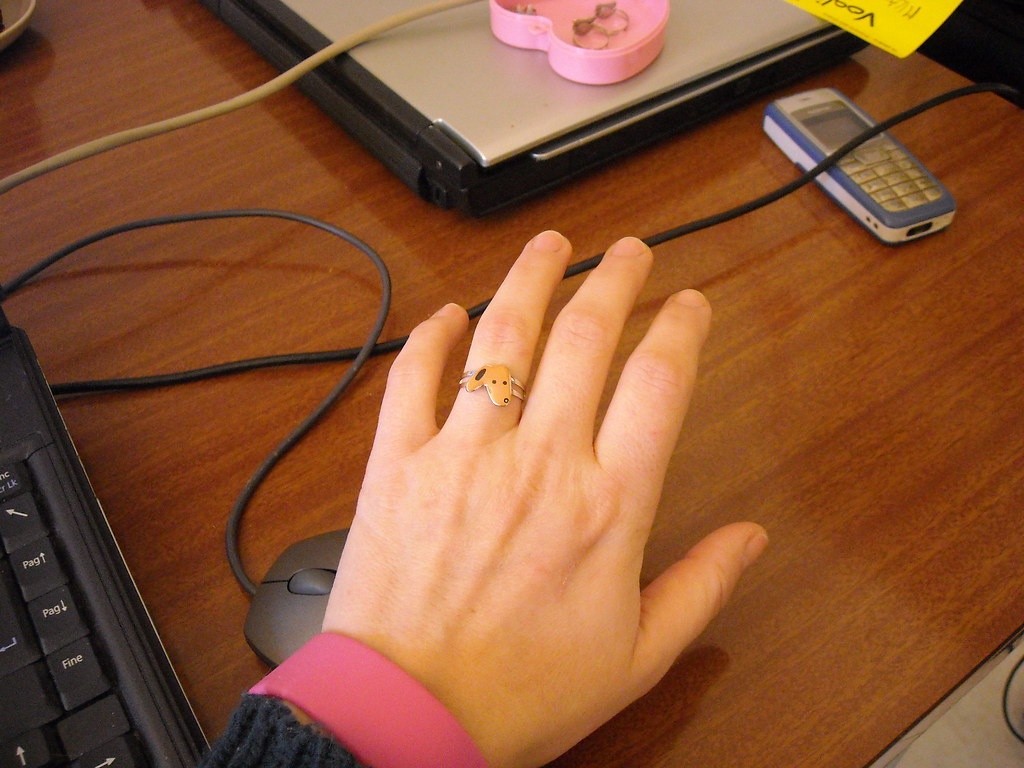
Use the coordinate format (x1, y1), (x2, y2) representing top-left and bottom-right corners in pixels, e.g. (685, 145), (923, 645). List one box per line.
(764, 87), (957, 247)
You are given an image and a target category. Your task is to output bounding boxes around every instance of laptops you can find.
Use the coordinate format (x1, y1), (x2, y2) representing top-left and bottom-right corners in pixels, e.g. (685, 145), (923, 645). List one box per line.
(201, 0), (871, 216)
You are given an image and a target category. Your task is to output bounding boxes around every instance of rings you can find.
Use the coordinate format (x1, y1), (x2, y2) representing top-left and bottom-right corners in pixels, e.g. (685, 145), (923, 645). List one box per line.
(509, 4), (537, 15)
(572, 2), (630, 49)
(459, 366), (527, 406)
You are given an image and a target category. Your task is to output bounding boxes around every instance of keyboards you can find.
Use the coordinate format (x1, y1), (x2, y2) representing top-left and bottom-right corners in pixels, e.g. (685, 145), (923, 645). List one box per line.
(0, 309), (213, 768)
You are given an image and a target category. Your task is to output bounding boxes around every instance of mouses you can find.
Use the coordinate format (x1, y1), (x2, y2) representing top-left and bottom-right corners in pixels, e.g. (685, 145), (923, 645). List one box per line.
(243, 528), (351, 669)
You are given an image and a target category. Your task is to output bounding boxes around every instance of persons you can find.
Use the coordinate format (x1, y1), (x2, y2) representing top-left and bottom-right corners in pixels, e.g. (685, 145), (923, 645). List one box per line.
(200, 229), (768, 768)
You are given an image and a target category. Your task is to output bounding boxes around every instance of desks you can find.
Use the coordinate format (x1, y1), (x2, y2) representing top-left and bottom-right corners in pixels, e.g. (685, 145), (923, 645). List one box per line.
(0, 0), (1024, 768)
